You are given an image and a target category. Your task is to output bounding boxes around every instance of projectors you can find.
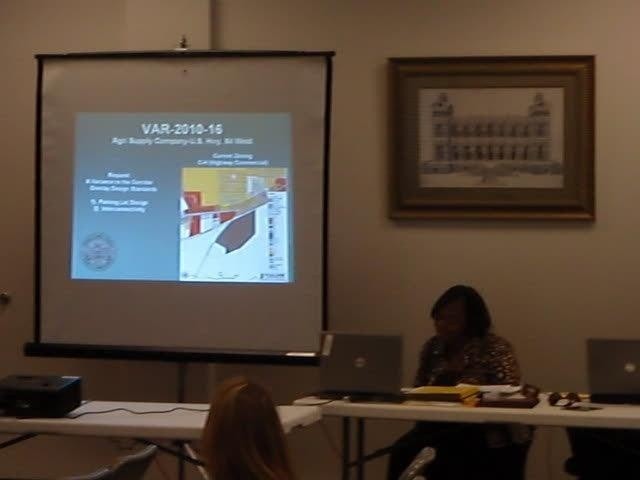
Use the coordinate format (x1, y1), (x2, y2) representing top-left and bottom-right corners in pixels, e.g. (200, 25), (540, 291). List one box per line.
(0, 374), (82, 418)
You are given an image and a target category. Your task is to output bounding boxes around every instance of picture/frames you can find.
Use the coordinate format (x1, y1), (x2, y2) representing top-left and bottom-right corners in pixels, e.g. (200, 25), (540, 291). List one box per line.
(382, 54), (597, 227)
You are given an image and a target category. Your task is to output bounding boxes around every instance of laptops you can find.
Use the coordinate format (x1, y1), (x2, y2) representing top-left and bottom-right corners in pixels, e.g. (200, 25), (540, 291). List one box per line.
(586, 339), (640, 403)
(317, 331), (412, 400)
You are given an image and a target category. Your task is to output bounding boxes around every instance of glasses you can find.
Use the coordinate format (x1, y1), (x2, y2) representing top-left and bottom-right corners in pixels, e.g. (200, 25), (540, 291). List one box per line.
(549, 393), (582, 410)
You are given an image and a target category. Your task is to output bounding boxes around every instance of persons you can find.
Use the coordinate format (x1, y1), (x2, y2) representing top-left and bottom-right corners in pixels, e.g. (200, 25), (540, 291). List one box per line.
(202, 376), (293, 480)
(386, 286), (532, 480)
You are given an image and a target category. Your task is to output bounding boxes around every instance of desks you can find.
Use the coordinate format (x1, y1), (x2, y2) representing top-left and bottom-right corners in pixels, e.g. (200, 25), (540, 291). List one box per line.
(4, 399), (321, 479)
(293, 390), (639, 478)
